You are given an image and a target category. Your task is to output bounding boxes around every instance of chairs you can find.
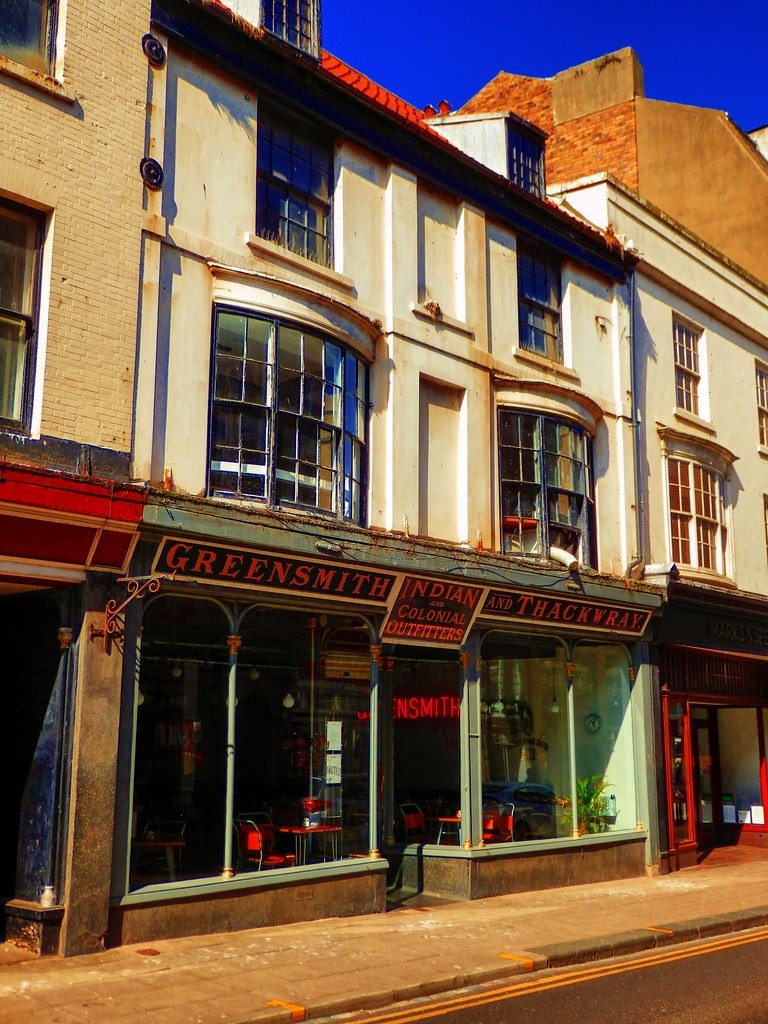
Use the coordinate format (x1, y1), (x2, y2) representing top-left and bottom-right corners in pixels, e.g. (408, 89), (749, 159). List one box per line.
(399, 803), (435, 845)
(233, 812), (296, 874)
(482, 803), (515, 844)
(144, 820), (187, 881)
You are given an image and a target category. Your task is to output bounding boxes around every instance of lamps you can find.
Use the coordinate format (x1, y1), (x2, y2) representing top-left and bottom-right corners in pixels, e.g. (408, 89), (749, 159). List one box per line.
(480, 660), (504, 711)
(247, 666), (260, 680)
(550, 657), (559, 713)
(170, 665), (182, 678)
(282, 688), (295, 708)
(137, 690), (145, 706)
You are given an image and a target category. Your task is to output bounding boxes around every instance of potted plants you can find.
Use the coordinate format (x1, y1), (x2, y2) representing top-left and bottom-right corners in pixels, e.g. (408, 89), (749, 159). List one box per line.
(560, 773), (613, 837)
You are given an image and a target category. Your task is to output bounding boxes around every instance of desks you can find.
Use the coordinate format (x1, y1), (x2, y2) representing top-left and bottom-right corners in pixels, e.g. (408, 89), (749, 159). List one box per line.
(437, 817), (462, 846)
(130, 840), (186, 887)
(275, 825), (346, 868)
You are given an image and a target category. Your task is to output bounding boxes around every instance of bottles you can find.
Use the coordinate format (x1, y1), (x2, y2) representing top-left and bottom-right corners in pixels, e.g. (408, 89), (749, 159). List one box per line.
(40, 886), (56, 907)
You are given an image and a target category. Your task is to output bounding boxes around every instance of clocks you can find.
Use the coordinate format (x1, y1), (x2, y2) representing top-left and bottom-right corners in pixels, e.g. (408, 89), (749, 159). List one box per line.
(584, 714), (603, 735)
